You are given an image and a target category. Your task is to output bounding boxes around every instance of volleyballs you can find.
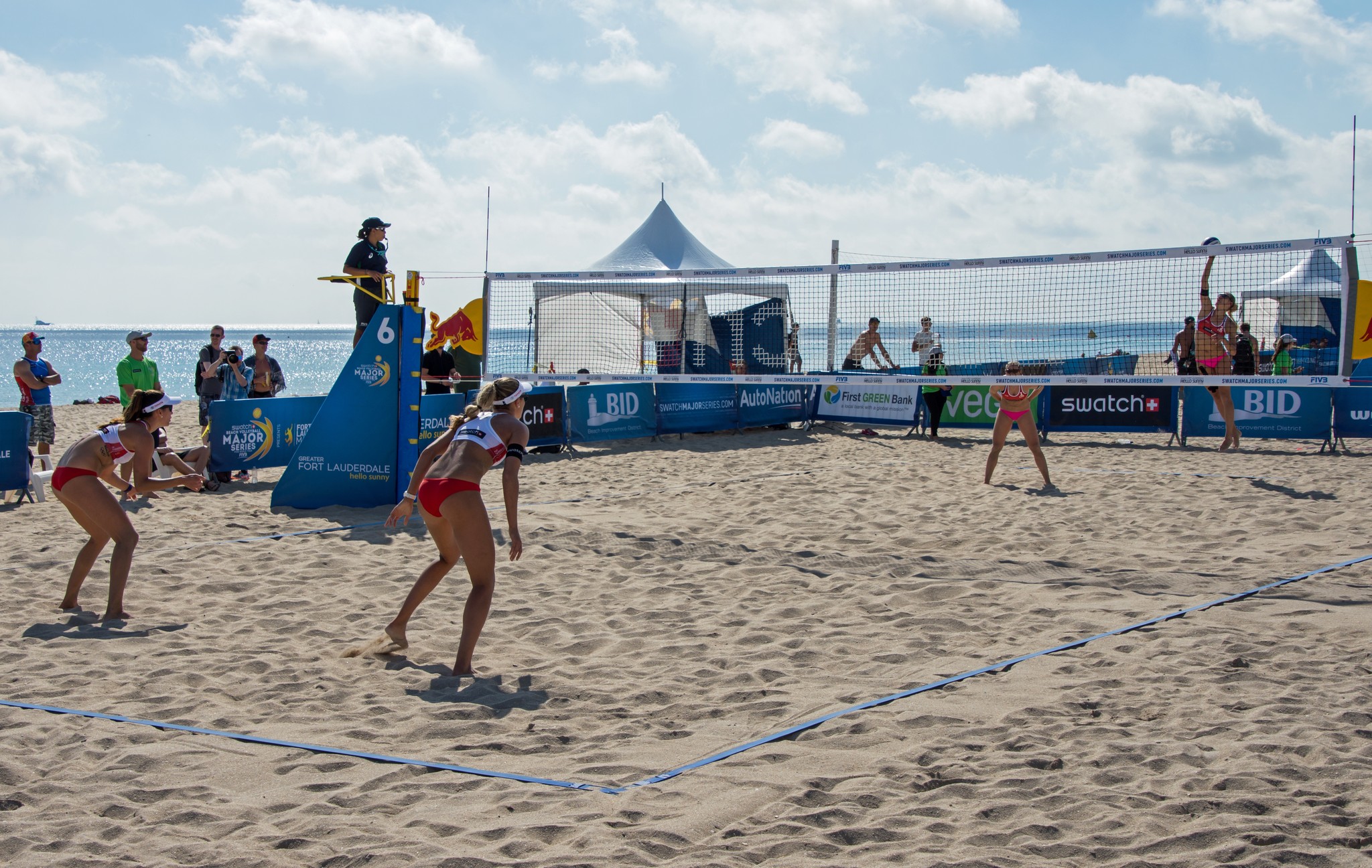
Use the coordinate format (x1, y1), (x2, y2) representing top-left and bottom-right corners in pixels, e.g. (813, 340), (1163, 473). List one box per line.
(1201, 237), (1220, 245)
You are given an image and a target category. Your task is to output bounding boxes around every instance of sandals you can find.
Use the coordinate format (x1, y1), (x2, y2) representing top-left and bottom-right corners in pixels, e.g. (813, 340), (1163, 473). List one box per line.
(184, 486), (205, 492)
(203, 480), (220, 491)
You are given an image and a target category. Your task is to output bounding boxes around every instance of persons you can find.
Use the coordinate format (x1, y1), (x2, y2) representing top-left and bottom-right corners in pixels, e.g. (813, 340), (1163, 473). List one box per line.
(343, 217), (395, 350)
(196, 325), (229, 446)
(735, 360), (747, 375)
(577, 369), (591, 386)
(246, 334), (287, 399)
(1163, 316), (1331, 376)
(52, 389), (207, 623)
(421, 333), (462, 395)
(207, 345), (254, 479)
(984, 360), (1055, 487)
(1194, 255), (1242, 452)
(911, 317), (954, 439)
(385, 376), (529, 677)
(116, 330), (164, 501)
(13, 332), (62, 479)
(788, 322), (802, 373)
(151, 427), (221, 493)
(842, 317), (900, 370)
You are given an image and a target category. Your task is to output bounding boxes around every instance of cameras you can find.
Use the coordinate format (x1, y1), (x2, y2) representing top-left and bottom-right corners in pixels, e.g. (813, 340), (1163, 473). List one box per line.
(223, 350), (239, 364)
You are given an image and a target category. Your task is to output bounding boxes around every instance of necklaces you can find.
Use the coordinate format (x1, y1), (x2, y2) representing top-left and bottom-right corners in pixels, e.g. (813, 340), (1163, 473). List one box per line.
(257, 357), (266, 364)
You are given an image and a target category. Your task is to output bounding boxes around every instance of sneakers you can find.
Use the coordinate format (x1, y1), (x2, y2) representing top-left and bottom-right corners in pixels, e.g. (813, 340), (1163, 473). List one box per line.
(231, 470), (250, 480)
(861, 428), (879, 436)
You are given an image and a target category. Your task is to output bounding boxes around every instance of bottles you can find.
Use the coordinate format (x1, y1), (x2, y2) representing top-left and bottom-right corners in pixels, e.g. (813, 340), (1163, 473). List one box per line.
(251, 466), (258, 484)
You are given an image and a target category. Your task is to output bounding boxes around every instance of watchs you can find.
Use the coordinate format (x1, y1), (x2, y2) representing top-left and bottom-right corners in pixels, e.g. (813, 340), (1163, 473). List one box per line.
(39, 375), (44, 382)
(439, 379), (443, 385)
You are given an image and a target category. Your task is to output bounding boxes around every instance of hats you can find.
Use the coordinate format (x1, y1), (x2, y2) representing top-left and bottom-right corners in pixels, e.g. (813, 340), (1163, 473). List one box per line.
(869, 318), (880, 324)
(492, 378), (532, 405)
(1184, 316), (1195, 324)
(142, 392), (182, 413)
(253, 334), (271, 344)
(22, 332), (45, 346)
(929, 347), (947, 356)
(1280, 334), (1297, 343)
(126, 330), (152, 344)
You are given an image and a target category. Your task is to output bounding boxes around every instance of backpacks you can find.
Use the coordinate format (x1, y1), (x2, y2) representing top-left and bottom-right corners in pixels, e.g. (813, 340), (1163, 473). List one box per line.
(1236, 334), (1255, 366)
(194, 344), (213, 396)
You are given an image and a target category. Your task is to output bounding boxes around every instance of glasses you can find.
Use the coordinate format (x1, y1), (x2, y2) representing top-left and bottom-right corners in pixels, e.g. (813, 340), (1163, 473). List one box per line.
(1218, 294), (1230, 299)
(163, 406), (173, 412)
(520, 394), (528, 402)
(1315, 342), (1318, 345)
(1324, 342), (1328, 344)
(255, 341), (268, 345)
(211, 334), (220, 337)
(238, 355), (242, 360)
(871, 322), (879, 326)
(134, 337), (147, 341)
(27, 341), (40, 345)
(1005, 369), (1018, 373)
(925, 323), (932, 326)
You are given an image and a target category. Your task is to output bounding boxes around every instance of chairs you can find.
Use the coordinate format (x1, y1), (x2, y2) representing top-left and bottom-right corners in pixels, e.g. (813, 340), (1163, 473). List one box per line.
(0, 411), (35, 504)
(150, 450), (210, 492)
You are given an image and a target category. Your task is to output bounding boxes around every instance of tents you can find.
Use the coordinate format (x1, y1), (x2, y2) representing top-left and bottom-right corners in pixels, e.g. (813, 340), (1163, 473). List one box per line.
(1239, 248), (1342, 351)
(536, 200), (790, 374)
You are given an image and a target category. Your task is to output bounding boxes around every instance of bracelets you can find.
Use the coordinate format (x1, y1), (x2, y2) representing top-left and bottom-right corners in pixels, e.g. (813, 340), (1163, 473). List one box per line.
(218, 358), (222, 362)
(403, 490), (417, 501)
(124, 483), (133, 493)
(1292, 370), (1294, 374)
